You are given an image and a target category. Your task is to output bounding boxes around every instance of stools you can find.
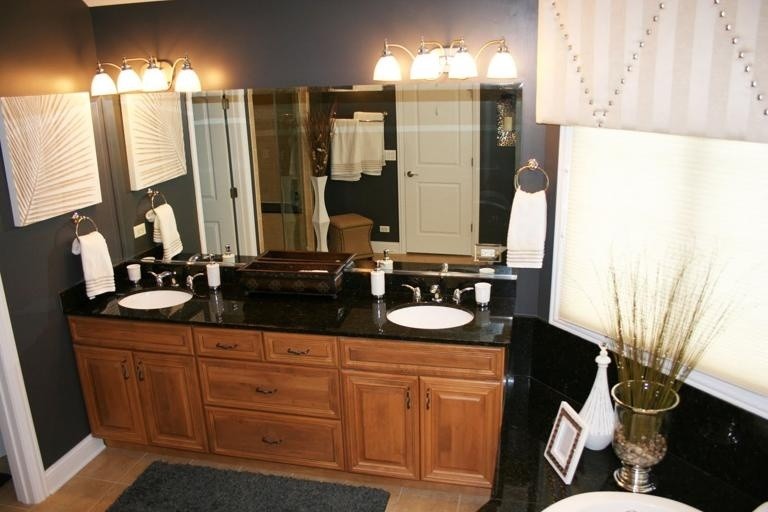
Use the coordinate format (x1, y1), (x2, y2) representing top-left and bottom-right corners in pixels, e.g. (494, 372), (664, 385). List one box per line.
(329, 213), (374, 260)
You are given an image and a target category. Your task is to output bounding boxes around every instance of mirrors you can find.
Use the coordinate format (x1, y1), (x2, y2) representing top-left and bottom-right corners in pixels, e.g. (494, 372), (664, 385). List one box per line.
(102, 81), (522, 281)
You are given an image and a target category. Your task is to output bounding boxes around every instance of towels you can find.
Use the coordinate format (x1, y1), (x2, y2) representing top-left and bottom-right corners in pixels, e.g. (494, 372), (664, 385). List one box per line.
(145, 202), (183, 263)
(327, 111), (362, 182)
(353, 111), (387, 177)
(72, 231), (116, 301)
(507, 187), (547, 269)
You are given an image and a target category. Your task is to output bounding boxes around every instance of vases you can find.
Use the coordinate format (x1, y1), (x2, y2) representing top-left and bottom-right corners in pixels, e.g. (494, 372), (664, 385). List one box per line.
(611, 380), (680, 496)
(309, 175), (330, 253)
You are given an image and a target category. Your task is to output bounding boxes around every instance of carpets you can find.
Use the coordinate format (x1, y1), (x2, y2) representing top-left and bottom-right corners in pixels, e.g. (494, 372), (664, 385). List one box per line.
(105, 460), (391, 512)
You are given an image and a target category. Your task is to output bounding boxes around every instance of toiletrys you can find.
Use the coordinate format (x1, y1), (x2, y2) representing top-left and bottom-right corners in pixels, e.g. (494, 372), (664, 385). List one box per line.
(206, 254), (221, 290)
(221, 245), (235, 264)
(370, 261), (386, 303)
(380, 249), (394, 269)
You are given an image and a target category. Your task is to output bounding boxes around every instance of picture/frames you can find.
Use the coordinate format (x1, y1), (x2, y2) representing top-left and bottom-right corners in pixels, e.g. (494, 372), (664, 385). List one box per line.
(543, 401), (589, 486)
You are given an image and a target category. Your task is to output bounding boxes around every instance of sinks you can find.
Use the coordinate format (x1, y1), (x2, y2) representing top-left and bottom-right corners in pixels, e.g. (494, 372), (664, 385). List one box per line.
(117, 288), (193, 310)
(386, 302), (474, 329)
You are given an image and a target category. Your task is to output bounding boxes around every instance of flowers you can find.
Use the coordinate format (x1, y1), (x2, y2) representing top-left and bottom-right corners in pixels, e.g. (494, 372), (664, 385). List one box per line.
(306, 110), (336, 176)
(600, 249), (733, 408)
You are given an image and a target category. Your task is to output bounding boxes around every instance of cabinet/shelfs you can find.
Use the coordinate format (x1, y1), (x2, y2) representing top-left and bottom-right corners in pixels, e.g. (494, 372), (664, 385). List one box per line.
(66, 315), (210, 454)
(335, 336), (506, 490)
(193, 325), (346, 473)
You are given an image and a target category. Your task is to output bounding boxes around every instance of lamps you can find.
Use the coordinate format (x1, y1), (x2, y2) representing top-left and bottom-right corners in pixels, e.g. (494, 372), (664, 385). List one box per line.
(91, 0), (201, 97)
(373, 0), (518, 81)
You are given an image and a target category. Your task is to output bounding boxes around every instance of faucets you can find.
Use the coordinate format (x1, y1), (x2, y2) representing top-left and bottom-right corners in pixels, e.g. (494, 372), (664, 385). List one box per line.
(440, 263), (449, 272)
(188, 253), (201, 262)
(429, 284), (444, 302)
(169, 271), (180, 288)
